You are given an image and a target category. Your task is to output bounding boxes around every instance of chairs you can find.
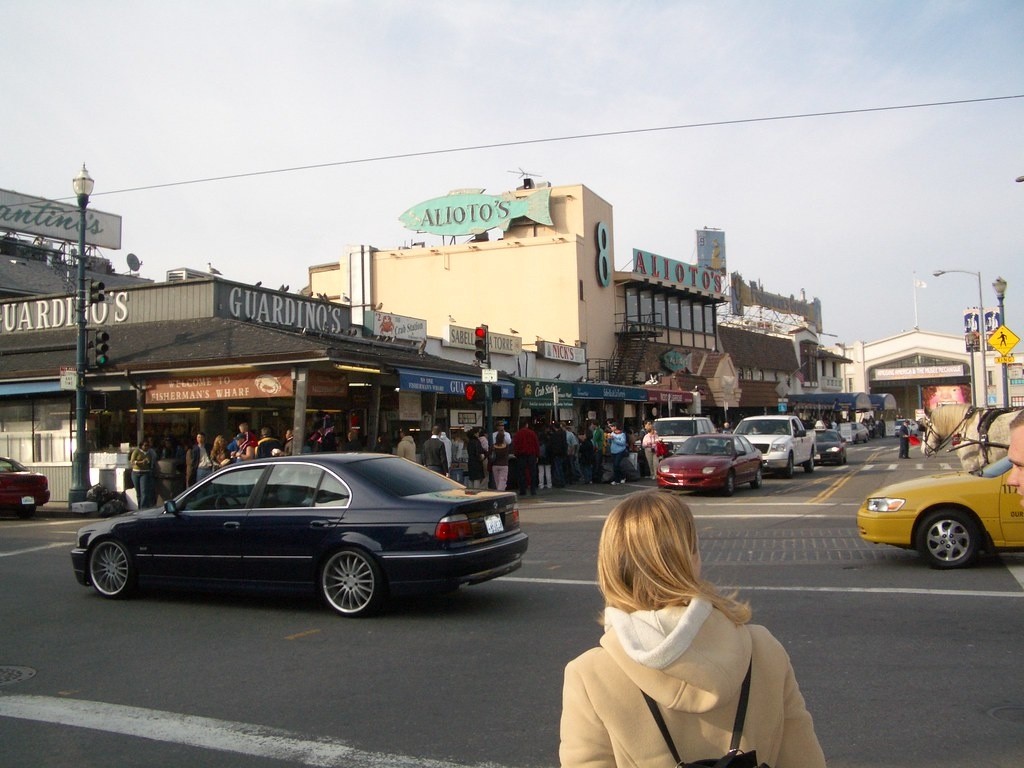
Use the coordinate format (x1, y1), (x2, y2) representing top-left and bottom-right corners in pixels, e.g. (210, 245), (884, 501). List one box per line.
(680, 429), (692, 435)
(278, 481), (307, 508)
(696, 443), (708, 453)
(318, 478), (344, 502)
(725, 442), (732, 454)
(663, 426), (674, 435)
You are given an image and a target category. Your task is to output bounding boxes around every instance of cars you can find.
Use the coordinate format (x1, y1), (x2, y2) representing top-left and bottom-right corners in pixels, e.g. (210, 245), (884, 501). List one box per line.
(0, 456), (50, 518)
(857, 456), (1024, 570)
(813, 429), (846, 465)
(634, 417), (719, 454)
(895, 419), (919, 437)
(70, 452), (529, 618)
(657, 434), (763, 496)
(837, 422), (869, 445)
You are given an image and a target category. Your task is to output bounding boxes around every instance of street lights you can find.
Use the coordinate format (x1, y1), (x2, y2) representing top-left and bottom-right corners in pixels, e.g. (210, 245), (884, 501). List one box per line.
(933, 269), (988, 407)
(992, 276), (1009, 409)
(69, 162), (95, 509)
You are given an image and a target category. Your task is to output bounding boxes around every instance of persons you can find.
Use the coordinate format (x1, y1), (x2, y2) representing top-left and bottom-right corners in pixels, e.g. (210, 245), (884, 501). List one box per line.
(128, 422), (393, 510)
(421, 418), (660, 497)
(862, 416), (885, 438)
(722, 421), (733, 434)
(398, 428), (416, 463)
(899, 420), (910, 459)
(823, 414), (843, 428)
(802, 419), (814, 429)
(750, 425), (761, 434)
(559, 489), (826, 768)
(1007, 411), (1024, 506)
(895, 415), (903, 420)
(774, 423), (786, 434)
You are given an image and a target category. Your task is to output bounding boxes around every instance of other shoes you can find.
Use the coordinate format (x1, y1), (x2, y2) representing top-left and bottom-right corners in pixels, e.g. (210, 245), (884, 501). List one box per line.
(621, 479), (626, 483)
(519, 491), (526, 496)
(531, 491), (537, 495)
(905, 457), (910, 459)
(899, 457), (904, 459)
(611, 482), (618, 484)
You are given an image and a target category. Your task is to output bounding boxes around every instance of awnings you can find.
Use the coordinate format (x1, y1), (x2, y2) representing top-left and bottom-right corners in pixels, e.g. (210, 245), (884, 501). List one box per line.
(785, 392), (897, 410)
(570, 380), (693, 404)
(380, 369), (515, 398)
(509, 375), (574, 408)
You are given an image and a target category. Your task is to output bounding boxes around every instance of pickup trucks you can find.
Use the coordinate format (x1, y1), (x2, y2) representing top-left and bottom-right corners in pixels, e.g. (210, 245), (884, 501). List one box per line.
(723, 415), (817, 479)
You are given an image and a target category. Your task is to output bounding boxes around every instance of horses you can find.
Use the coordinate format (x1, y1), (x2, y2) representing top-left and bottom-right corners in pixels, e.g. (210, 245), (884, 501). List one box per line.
(920, 403), (1024, 471)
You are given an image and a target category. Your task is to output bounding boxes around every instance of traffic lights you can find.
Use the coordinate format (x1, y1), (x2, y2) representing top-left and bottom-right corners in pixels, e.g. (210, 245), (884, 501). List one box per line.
(474, 326), (486, 360)
(88, 280), (105, 303)
(465, 384), (478, 401)
(88, 330), (109, 366)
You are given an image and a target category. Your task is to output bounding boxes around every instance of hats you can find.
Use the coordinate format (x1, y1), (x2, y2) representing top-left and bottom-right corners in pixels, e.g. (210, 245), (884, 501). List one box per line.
(260, 428), (271, 437)
(612, 422), (618, 425)
(233, 433), (246, 440)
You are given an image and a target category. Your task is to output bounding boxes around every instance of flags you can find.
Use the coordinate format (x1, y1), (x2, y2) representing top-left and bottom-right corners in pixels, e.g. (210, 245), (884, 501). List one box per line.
(794, 366), (808, 384)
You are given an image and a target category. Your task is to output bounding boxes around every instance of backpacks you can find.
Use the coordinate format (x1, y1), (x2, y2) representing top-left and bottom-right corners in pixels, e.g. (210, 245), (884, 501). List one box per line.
(640, 658), (772, 768)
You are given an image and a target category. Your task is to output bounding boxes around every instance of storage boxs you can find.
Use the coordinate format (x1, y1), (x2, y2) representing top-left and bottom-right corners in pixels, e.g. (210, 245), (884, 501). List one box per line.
(72, 502), (98, 513)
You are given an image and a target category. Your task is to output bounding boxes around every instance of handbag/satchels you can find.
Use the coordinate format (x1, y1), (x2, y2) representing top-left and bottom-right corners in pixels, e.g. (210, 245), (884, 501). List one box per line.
(656, 441), (668, 457)
(488, 452), (496, 462)
